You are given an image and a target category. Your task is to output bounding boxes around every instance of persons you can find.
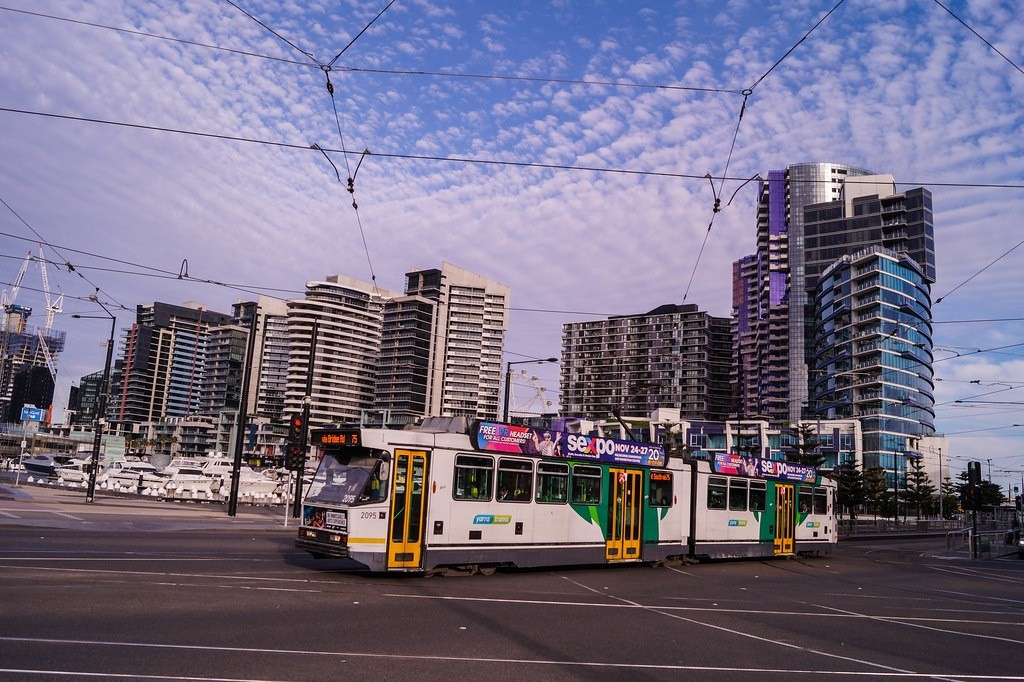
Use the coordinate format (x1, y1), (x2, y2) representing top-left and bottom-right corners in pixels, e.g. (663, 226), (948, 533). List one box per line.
(801, 503), (808, 514)
(498, 484), (511, 500)
(1011, 516), (1021, 545)
(743, 457), (761, 477)
(660, 495), (670, 505)
(849, 507), (859, 531)
(532, 430), (564, 456)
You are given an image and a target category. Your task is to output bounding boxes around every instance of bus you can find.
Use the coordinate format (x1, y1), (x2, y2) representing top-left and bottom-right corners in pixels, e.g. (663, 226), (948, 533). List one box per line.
(294, 415), (839, 579)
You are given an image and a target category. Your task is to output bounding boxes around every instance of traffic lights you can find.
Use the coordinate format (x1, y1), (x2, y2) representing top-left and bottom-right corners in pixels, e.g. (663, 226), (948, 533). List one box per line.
(960, 486), (970, 511)
(286, 444), (303, 470)
(289, 414), (303, 442)
(968, 485), (982, 509)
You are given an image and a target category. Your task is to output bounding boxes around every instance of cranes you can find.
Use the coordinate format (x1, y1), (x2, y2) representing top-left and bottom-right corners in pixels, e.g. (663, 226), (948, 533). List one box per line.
(1, 243), (65, 335)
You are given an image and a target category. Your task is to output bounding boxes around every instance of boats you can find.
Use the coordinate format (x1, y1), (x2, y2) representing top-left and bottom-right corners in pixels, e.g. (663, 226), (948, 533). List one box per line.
(0, 445), (346, 503)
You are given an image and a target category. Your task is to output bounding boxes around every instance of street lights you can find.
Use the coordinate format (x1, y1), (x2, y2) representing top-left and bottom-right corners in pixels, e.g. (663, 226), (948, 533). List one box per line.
(938, 447), (943, 521)
(503, 357), (559, 422)
(894, 442), (906, 531)
(70, 314), (117, 503)
